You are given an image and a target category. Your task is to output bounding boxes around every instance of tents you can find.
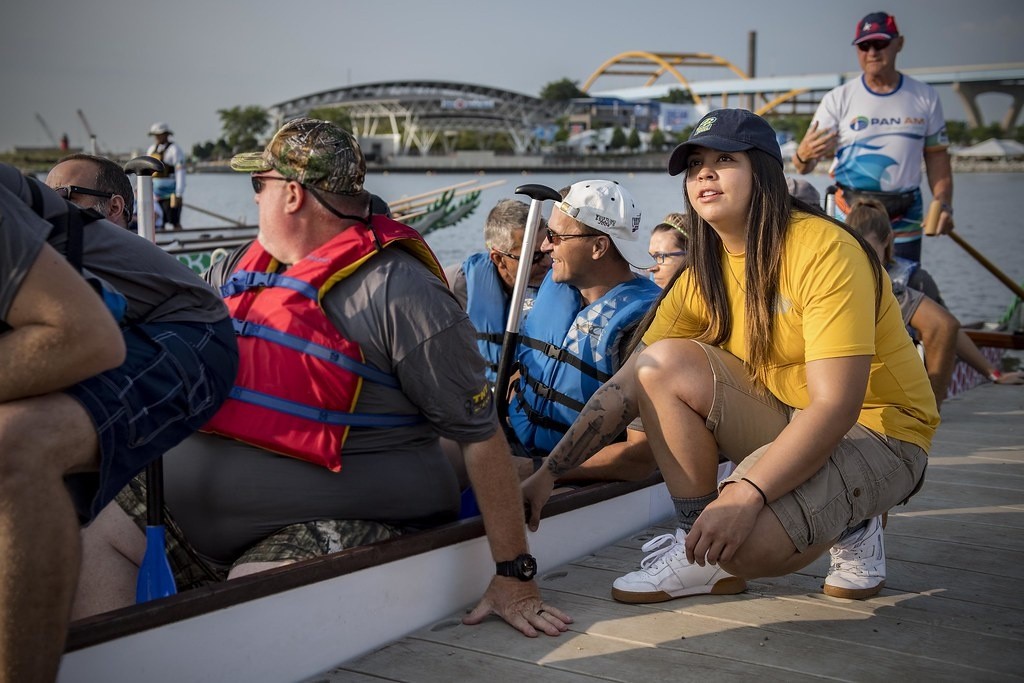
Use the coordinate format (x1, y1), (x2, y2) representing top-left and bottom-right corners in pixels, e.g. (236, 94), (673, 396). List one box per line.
(780, 140), (801, 159)
(565, 126), (679, 147)
(956, 138), (1024, 156)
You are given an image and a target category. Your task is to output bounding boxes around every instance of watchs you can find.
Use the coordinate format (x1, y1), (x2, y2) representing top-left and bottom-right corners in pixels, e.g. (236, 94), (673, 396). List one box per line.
(496, 554), (538, 583)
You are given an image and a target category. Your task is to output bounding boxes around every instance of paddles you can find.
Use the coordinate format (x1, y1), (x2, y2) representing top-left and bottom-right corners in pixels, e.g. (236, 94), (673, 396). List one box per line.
(461, 183), (564, 521)
(177, 193), (248, 230)
(924, 201), (1024, 302)
(122, 155), (179, 603)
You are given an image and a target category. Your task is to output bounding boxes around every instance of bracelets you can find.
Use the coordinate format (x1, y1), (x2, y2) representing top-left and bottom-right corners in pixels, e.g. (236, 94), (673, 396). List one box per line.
(741, 477), (768, 505)
(796, 151), (809, 164)
(532, 457), (544, 473)
(987, 369), (1004, 383)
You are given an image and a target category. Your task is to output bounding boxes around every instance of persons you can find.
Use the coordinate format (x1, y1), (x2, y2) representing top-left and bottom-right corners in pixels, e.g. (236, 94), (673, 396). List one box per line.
(68, 118), (573, 637)
(506, 178), (666, 481)
(132, 122), (185, 234)
(844, 197), (1024, 386)
(521, 108), (941, 605)
(0, 163), (239, 683)
(46, 154), (133, 234)
(454, 199), (553, 392)
(649, 212), (695, 290)
(787, 178), (820, 210)
(889, 281), (961, 414)
(792, 11), (954, 269)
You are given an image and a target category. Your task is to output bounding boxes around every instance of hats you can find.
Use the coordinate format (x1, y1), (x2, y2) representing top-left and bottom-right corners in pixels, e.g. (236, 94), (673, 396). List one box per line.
(554, 179), (655, 269)
(852, 12), (899, 46)
(665, 214), (694, 239)
(148, 123), (174, 135)
(668, 109), (783, 176)
(231, 118), (366, 195)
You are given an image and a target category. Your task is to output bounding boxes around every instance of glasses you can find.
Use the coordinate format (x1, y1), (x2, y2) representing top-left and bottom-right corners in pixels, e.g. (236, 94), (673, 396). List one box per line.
(251, 177), (308, 194)
(55, 186), (112, 199)
(650, 252), (688, 265)
(546, 229), (602, 244)
(492, 247), (545, 265)
(857, 36), (896, 51)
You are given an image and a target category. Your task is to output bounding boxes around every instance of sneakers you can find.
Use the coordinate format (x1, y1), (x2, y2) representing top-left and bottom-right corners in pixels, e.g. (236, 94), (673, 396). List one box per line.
(824, 512), (888, 598)
(611, 528), (746, 603)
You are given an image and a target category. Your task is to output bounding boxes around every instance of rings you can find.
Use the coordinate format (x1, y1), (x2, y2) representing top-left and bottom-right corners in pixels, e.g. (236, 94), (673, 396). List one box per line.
(536, 609), (545, 615)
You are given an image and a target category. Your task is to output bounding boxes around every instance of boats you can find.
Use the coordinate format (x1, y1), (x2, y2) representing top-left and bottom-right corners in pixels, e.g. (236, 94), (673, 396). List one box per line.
(131, 176), (511, 275)
(56, 291), (1024, 683)
